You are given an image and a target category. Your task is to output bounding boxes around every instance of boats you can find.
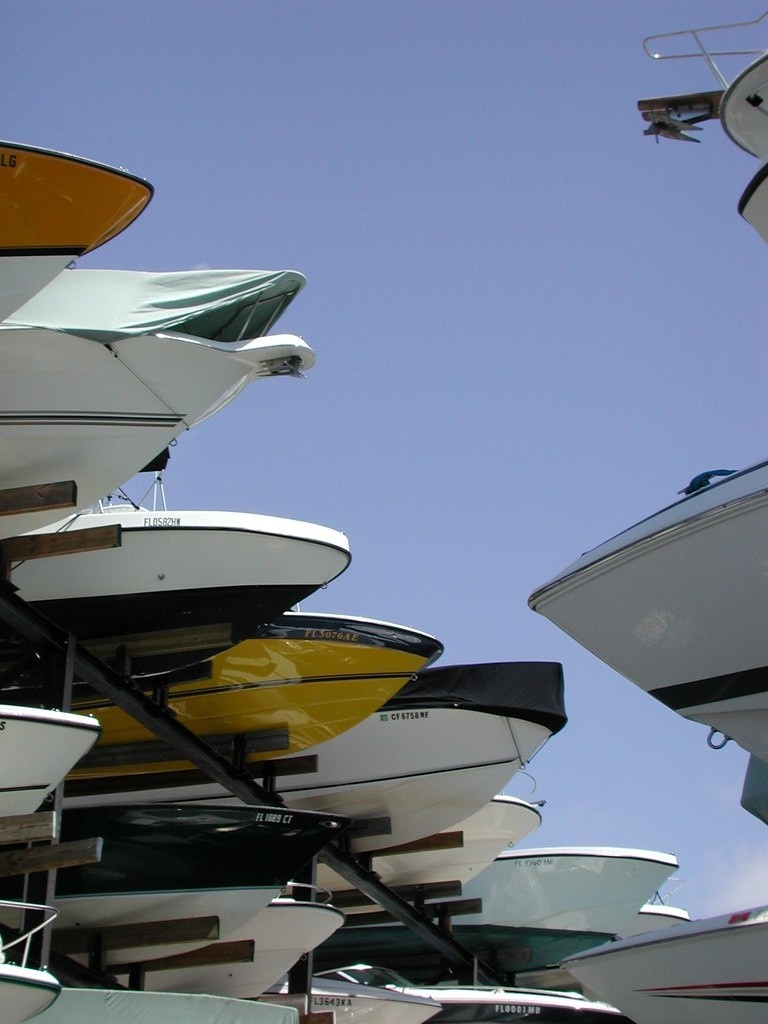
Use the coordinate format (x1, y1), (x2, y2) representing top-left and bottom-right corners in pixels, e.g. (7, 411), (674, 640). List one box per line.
(2, 12), (768, 1024)
